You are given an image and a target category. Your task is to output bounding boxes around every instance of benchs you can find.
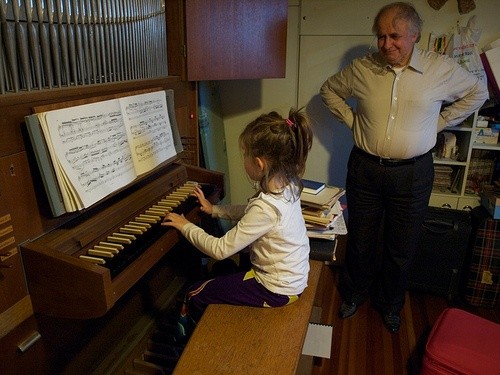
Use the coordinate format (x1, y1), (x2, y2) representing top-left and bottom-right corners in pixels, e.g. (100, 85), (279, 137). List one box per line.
(171, 258), (324, 375)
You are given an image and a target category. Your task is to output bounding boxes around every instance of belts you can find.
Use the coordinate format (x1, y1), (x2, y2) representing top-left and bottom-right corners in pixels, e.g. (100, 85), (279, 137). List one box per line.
(353, 145), (432, 168)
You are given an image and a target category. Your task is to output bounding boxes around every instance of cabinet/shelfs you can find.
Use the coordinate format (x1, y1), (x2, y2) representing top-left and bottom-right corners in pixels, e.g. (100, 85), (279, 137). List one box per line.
(165, 0), (288, 78)
(427, 108), (500, 213)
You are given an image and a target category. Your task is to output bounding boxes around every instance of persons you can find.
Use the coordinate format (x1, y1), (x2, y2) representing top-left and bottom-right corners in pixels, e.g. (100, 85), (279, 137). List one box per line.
(320, 3), (489, 333)
(160, 106), (313, 345)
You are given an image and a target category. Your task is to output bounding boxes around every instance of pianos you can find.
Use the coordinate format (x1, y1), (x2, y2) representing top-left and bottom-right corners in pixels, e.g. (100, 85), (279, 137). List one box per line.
(0, 76), (246, 375)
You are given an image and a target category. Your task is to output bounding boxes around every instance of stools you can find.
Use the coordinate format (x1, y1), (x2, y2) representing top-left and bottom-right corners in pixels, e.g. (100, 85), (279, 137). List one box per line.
(418, 307), (500, 375)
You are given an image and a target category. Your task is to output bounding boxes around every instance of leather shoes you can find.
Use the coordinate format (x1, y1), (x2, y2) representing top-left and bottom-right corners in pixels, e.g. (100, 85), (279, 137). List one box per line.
(383, 310), (401, 334)
(336, 287), (358, 319)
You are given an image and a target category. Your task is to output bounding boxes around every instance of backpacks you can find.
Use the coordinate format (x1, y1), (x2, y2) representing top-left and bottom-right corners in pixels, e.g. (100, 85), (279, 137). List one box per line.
(455, 206), (500, 295)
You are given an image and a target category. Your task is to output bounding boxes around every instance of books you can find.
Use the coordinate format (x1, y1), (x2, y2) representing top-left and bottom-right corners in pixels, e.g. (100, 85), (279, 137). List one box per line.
(38, 90), (177, 213)
(300, 179), (348, 262)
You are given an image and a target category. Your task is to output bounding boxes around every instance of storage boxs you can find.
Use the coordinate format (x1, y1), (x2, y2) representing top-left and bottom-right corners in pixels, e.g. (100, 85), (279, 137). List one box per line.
(480, 189), (500, 220)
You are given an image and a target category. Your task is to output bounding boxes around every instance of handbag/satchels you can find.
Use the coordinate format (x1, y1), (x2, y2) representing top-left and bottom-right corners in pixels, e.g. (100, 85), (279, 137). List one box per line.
(444, 33), (489, 98)
